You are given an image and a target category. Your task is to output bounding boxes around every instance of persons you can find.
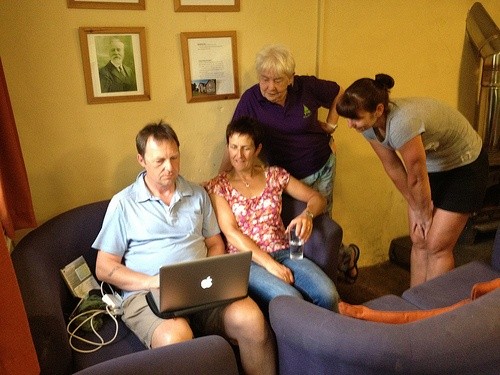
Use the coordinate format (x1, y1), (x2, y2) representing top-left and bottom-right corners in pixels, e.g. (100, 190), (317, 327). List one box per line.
(202, 118), (342, 316)
(336, 74), (487, 287)
(98, 38), (138, 92)
(90, 118), (276, 375)
(219, 47), (360, 283)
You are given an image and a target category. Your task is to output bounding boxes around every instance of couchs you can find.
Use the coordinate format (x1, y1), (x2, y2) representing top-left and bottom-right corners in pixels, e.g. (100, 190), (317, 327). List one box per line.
(268, 238), (500, 375)
(11, 194), (343, 375)
(72, 335), (238, 375)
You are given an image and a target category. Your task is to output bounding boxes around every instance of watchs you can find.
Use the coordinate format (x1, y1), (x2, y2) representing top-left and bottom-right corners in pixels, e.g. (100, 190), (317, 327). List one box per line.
(301, 210), (313, 218)
(325, 121), (338, 131)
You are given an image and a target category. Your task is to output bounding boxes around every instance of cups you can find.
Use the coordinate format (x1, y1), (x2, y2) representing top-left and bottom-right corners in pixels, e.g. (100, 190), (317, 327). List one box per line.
(288, 227), (303, 260)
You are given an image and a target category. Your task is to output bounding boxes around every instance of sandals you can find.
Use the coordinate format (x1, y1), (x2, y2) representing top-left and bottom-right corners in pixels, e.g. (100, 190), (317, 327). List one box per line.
(344, 244), (360, 283)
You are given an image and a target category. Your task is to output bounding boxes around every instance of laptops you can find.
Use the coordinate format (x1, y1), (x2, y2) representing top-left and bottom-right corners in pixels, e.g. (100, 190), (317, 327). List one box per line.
(149, 250), (252, 314)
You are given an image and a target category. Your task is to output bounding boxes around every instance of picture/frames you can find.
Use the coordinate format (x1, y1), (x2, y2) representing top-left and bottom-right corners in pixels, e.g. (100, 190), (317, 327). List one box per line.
(79, 27), (151, 105)
(67, 0), (145, 10)
(181, 31), (240, 103)
(174, 0), (240, 12)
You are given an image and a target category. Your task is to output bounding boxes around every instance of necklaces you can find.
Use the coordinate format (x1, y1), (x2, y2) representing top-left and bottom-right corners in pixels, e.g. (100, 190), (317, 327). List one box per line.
(246, 181), (250, 188)
